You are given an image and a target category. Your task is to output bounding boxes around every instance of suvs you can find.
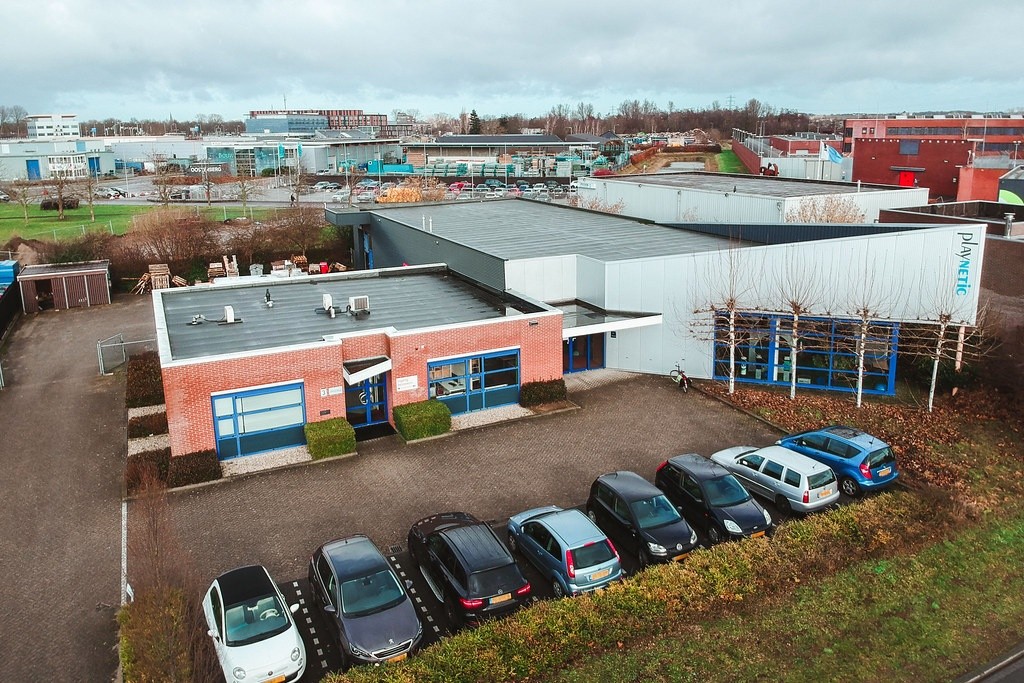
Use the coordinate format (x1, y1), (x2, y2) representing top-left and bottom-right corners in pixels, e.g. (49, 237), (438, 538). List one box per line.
(708, 443), (840, 517)
(654, 453), (773, 544)
(407, 510), (533, 636)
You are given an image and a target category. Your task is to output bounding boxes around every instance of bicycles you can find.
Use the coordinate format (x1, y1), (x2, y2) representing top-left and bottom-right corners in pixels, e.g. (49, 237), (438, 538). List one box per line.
(669, 357), (688, 393)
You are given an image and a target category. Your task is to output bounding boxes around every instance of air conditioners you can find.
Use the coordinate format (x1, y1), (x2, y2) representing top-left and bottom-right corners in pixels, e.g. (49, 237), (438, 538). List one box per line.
(224, 305), (234, 323)
(323, 293), (332, 310)
(349, 295), (369, 311)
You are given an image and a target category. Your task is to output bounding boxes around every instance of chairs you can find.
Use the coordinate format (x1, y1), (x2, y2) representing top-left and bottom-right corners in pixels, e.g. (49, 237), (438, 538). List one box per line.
(252, 597), (276, 621)
(226, 605), (248, 634)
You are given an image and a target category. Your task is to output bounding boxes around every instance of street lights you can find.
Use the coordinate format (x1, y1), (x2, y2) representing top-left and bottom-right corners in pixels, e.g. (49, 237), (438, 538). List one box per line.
(1013, 139), (1021, 167)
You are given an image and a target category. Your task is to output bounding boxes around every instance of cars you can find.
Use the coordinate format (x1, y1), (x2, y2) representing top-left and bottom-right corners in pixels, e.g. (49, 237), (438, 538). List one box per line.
(0, 189), (10, 202)
(310, 179), (579, 204)
(715, 314), (873, 381)
(774, 423), (900, 496)
(201, 563), (306, 683)
(584, 466), (698, 571)
(39, 184), (238, 209)
(505, 504), (624, 599)
(307, 533), (423, 668)
